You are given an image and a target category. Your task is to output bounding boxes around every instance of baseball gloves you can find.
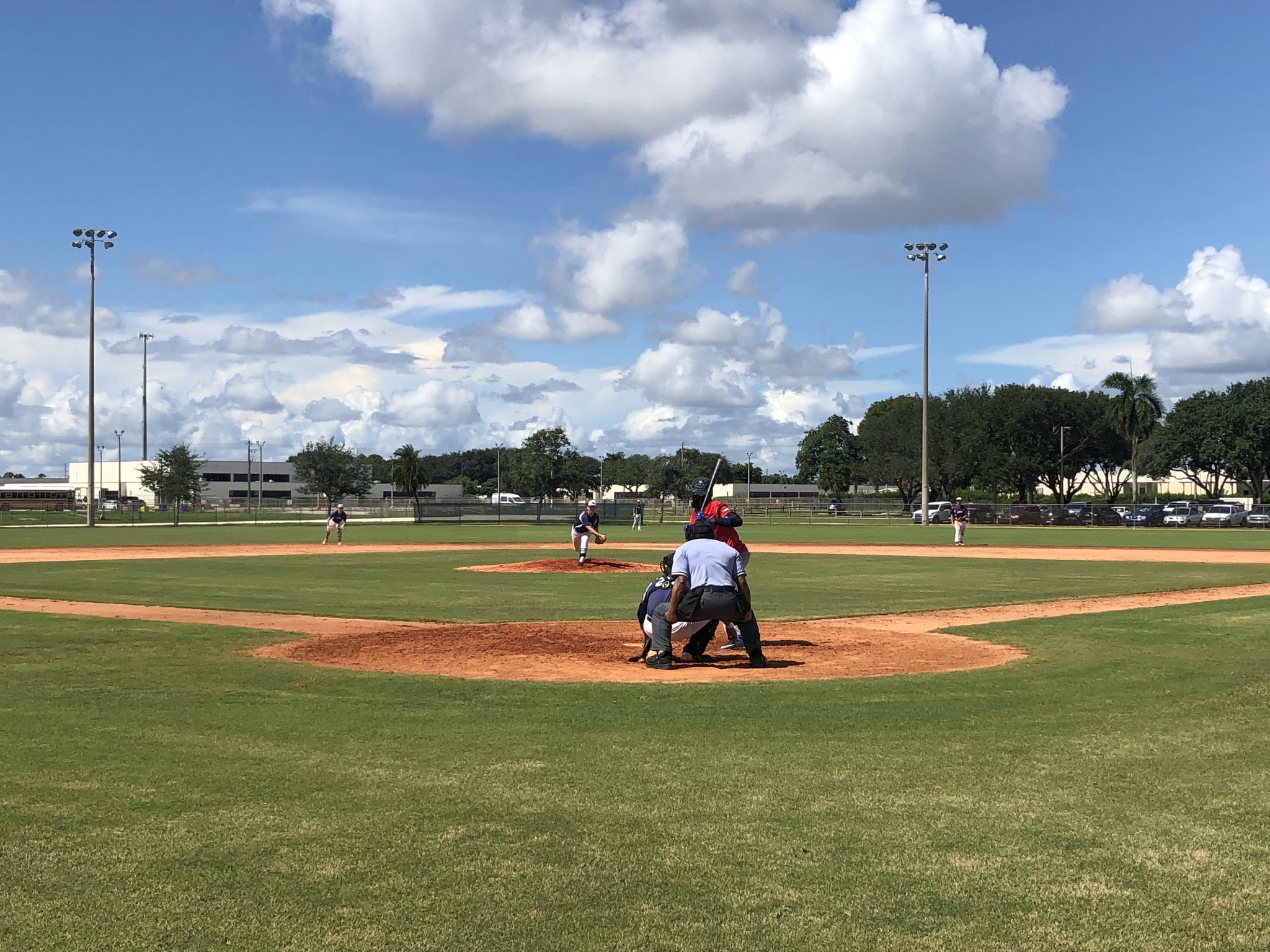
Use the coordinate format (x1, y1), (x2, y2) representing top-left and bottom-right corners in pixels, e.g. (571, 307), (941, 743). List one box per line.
(340, 526), (345, 532)
(595, 537), (606, 545)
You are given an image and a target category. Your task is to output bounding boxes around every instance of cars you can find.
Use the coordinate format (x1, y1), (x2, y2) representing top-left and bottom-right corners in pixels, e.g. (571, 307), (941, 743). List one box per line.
(1162, 500), (1199, 512)
(1079, 507), (1121, 526)
(828, 499), (846, 514)
(912, 501), (956, 524)
(1111, 507), (1131, 523)
(1040, 509), (1082, 526)
(998, 505), (1041, 525)
(963, 504), (997, 524)
(1037, 505), (1050, 514)
(1065, 502), (1093, 517)
(1163, 507), (1204, 528)
(101, 500), (122, 511)
(1247, 507), (1270, 529)
(1124, 505), (1170, 527)
(75, 498), (87, 510)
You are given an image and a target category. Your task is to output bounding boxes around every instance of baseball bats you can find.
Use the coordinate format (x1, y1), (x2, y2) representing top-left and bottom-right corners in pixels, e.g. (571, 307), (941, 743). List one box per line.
(700, 459), (723, 512)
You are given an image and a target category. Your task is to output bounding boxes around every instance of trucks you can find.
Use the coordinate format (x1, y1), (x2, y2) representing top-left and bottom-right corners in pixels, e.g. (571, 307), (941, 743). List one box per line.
(492, 493), (525, 504)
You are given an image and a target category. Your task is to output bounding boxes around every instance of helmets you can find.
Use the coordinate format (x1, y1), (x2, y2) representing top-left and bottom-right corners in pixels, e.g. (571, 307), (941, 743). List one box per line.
(660, 552), (674, 574)
(684, 520), (715, 541)
(691, 477), (713, 501)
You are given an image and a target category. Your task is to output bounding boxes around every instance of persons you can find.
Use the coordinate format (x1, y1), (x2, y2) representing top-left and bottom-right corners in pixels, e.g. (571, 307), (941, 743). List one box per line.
(632, 499), (642, 531)
(950, 497), (968, 546)
(637, 477), (767, 670)
(322, 504), (347, 545)
(571, 500), (607, 568)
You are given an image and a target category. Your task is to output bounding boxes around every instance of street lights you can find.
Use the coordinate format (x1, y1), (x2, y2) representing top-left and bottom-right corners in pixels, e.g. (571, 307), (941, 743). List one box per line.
(905, 242), (949, 526)
(72, 228), (118, 528)
(1060, 426), (1072, 509)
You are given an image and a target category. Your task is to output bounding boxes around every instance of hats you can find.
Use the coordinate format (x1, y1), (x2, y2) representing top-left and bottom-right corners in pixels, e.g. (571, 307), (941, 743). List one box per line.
(588, 500), (597, 507)
(338, 504), (343, 508)
(637, 500), (640, 502)
(956, 497), (962, 500)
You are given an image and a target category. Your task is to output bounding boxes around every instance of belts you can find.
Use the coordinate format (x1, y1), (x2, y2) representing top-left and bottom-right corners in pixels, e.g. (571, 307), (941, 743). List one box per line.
(573, 528), (580, 534)
(703, 586), (733, 593)
(647, 616), (652, 622)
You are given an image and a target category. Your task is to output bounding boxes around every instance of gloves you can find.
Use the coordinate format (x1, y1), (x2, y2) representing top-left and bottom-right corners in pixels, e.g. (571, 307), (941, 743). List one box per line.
(683, 524), (690, 531)
(326, 527), (331, 533)
(696, 512), (710, 522)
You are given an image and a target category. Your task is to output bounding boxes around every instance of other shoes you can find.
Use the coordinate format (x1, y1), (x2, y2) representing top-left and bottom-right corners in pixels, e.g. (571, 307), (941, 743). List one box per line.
(715, 635), (746, 649)
(577, 564), (583, 568)
(338, 542), (342, 545)
(639, 649), (657, 662)
(749, 654), (769, 666)
(681, 646), (717, 663)
(322, 538), (328, 545)
(638, 529), (642, 532)
(959, 541), (965, 545)
(645, 650), (673, 669)
(632, 528), (635, 531)
(955, 542), (959, 546)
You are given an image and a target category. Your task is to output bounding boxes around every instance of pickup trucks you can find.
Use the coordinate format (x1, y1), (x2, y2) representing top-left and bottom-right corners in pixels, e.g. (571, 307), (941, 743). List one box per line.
(1200, 504), (1248, 528)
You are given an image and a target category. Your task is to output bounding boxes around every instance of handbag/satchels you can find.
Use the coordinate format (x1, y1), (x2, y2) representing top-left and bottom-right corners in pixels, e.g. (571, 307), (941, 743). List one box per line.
(675, 587), (703, 618)
